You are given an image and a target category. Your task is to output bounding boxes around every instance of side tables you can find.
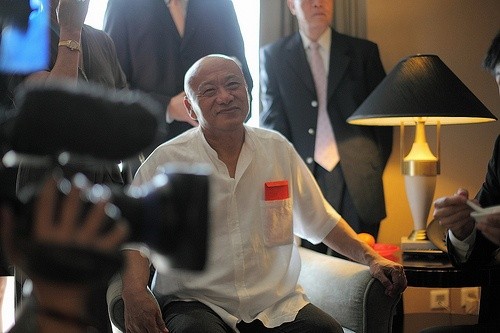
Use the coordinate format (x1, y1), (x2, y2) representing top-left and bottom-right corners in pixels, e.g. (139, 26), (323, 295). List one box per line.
(379, 242), (499, 333)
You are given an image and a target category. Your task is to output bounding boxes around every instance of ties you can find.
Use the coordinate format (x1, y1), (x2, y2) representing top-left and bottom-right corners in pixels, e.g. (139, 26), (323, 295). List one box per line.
(307, 40), (340, 172)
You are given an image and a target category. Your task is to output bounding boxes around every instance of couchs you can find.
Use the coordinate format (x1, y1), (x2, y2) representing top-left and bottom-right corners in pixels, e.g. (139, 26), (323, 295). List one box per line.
(107, 233), (405, 332)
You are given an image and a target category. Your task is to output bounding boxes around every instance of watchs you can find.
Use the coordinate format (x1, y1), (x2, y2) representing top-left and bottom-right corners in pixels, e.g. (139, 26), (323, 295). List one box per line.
(59, 38), (83, 52)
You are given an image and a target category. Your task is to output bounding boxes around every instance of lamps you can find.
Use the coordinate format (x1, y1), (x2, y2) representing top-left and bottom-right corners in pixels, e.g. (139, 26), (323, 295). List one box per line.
(345, 55), (497, 255)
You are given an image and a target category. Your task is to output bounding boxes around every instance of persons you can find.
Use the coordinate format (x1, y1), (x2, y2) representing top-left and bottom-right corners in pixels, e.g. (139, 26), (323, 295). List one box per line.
(104, 0), (252, 140)
(118, 53), (410, 333)
(429, 30), (500, 331)
(0, 167), (128, 332)
(2, 1), (132, 200)
(260, 0), (393, 261)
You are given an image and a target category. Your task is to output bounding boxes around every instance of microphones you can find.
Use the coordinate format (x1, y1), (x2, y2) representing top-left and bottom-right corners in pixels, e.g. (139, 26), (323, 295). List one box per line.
(0, 77), (161, 159)
